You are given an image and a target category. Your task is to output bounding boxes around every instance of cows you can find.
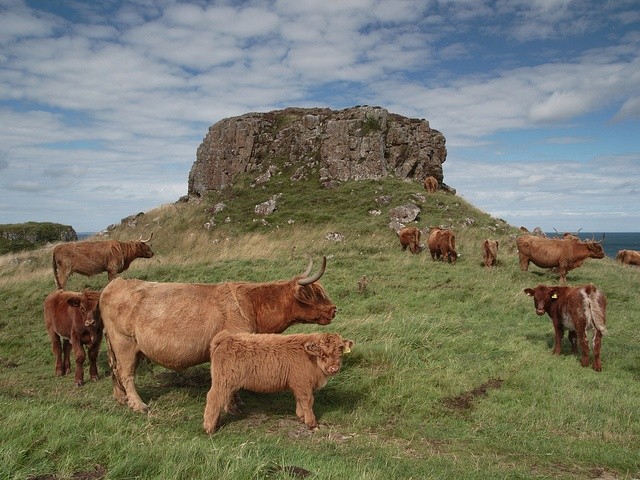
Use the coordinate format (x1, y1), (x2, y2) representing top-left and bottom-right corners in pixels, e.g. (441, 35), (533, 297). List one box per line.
(43, 288), (104, 388)
(52, 232), (155, 290)
(97, 254), (338, 415)
(517, 225), (606, 285)
(203, 329), (354, 435)
(482, 239), (500, 267)
(399, 227), (424, 255)
(524, 283), (609, 372)
(616, 249), (640, 265)
(428, 225), (461, 266)
(423, 176), (438, 193)
